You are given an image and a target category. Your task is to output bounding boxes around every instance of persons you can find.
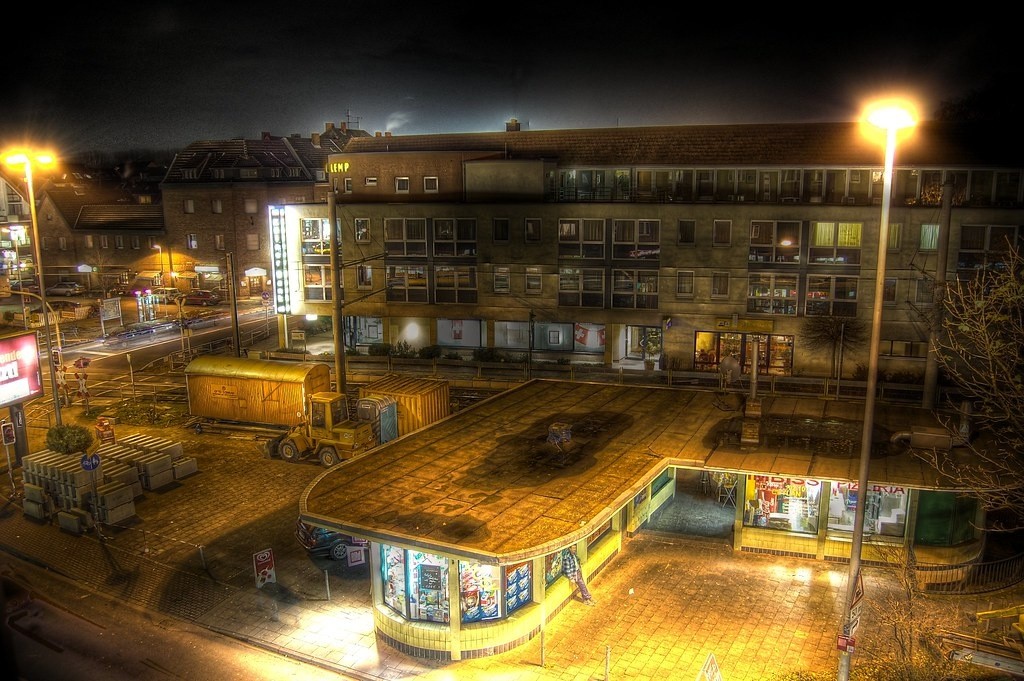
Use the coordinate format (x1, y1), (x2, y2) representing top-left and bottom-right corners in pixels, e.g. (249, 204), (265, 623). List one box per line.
(562, 544), (596, 605)
(699, 349), (709, 370)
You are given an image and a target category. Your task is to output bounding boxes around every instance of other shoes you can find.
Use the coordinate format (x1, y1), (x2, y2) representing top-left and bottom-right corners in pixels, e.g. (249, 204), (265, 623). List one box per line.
(583, 599), (594, 606)
(590, 598), (597, 603)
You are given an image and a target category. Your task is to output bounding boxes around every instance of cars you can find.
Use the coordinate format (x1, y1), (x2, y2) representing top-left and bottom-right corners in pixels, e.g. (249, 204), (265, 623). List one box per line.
(178, 290), (219, 307)
(293, 514), (370, 563)
(144, 287), (180, 304)
(10, 280), (40, 296)
(45, 281), (87, 298)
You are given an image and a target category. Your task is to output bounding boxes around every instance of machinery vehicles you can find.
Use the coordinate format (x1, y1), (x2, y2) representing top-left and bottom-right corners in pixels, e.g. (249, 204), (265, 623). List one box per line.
(268, 391), (379, 469)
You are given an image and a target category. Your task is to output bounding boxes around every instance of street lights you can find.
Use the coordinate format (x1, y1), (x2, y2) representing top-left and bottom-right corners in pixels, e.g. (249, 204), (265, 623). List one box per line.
(153, 244), (169, 317)
(837, 102), (917, 681)
(7, 152), (62, 428)
